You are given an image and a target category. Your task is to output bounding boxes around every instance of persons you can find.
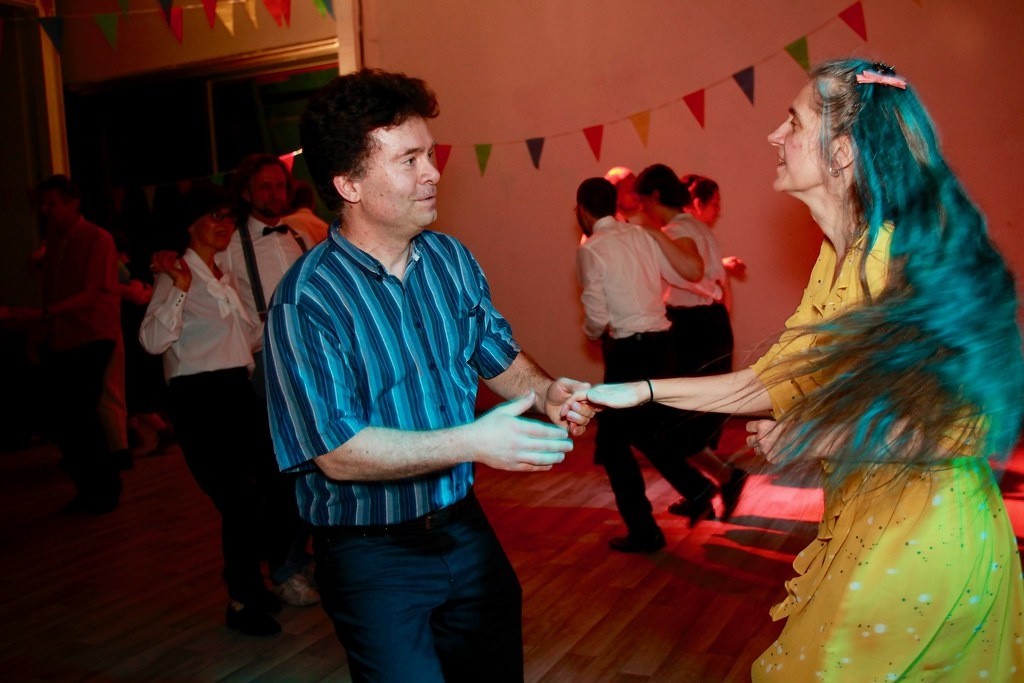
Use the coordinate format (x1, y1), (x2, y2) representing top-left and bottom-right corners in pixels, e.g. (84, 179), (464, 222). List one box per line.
(212, 153), (322, 608)
(277, 179), (331, 245)
(138, 187), (286, 637)
(9, 175), (134, 518)
(572, 178), (719, 554)
(564, 58), (1024, 682)
(260, 70), (599, 682)
(604, 162), (750, 525)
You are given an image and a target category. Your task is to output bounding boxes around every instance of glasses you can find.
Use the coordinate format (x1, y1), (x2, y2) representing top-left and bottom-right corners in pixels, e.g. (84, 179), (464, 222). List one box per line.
(211, 210), (239, 224)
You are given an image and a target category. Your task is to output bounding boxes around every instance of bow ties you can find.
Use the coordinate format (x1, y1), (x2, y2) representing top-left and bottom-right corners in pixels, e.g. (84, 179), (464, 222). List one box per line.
(262, 224), (289, 237)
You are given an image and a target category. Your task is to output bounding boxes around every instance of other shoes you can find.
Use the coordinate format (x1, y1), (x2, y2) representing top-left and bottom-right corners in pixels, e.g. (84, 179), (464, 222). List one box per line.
(719, 466), (749, 520)
(248, 582), (284, 613)
(272, 574), (320, 605)
(224, 602), (283, 635)
(687, 478), (719, 527)
(667, 497), (716, 521)
(608, 527), (668, 555)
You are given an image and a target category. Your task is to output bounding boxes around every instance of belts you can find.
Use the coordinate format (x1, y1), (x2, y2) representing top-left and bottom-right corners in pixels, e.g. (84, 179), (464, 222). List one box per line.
(314, 486), (475, 536)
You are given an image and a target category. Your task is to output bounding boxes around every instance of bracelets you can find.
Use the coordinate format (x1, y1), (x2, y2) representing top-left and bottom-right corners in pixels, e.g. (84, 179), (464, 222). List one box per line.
(644, 379), (654, 402)
(42, 306), (51, 321)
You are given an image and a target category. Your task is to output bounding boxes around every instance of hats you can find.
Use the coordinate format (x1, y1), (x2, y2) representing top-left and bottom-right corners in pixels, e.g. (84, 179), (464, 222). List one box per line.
(179, 179), (237, 230)
